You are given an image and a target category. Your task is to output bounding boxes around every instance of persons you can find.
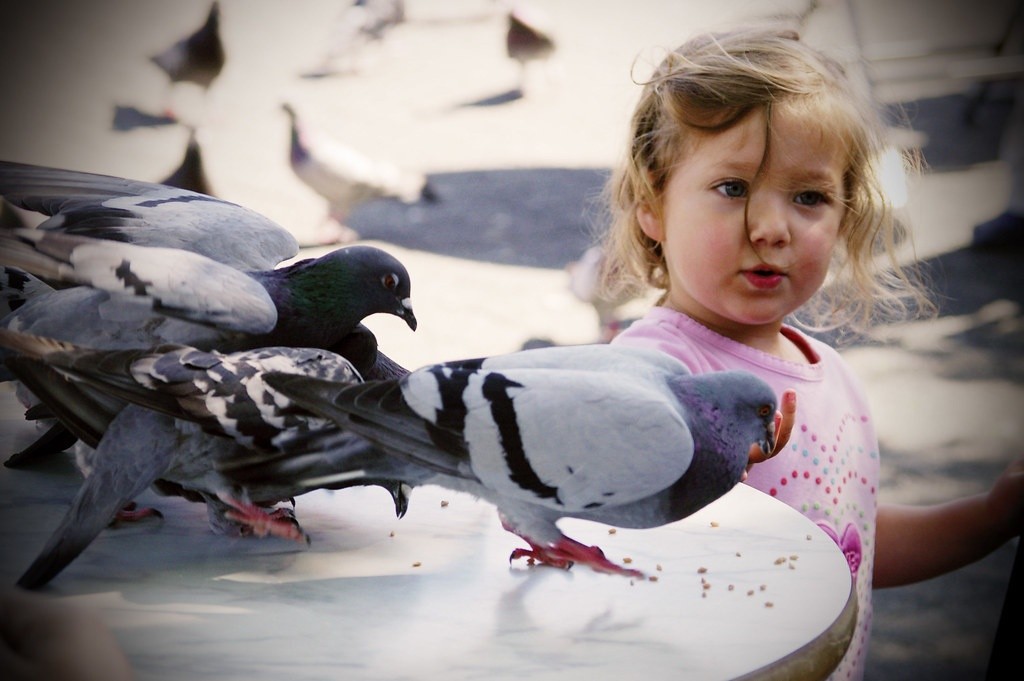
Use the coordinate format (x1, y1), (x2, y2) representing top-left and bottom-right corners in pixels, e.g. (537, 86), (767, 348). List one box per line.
(597, 29), (1024, 681)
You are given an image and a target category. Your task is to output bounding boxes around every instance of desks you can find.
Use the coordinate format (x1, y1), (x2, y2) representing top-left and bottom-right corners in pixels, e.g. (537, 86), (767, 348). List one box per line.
(0, 382), (859, 681)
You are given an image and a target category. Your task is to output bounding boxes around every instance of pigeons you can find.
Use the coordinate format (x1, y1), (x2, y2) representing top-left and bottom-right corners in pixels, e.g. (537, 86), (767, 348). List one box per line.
(0, 161), (419, 598)
(261, 343), (778, 576)
